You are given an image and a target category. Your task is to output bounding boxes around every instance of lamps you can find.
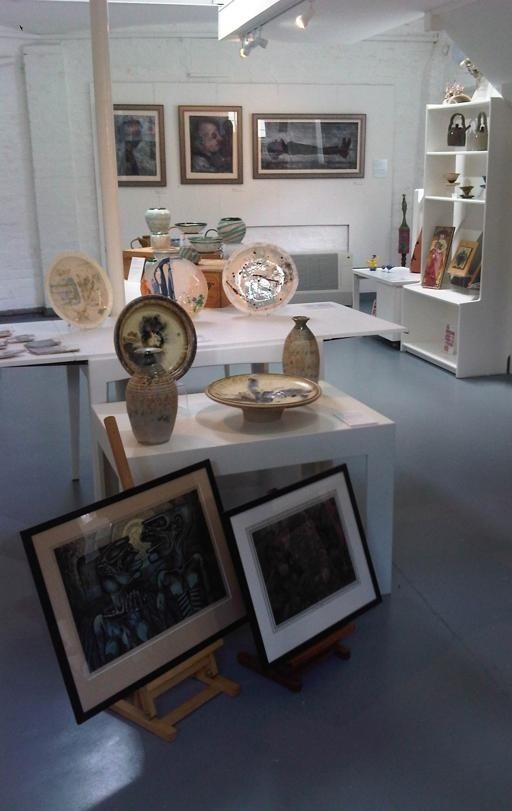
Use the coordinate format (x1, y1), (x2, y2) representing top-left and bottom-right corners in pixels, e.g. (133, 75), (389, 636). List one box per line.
(295, 0), (316, 29)
(239, 28), (268, 58)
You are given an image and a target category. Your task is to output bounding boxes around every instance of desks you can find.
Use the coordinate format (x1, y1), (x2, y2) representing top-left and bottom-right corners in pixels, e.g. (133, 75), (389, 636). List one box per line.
(0, 301), (408, 483)
(352, 267), (425, 342)
(141, 259), (232, 309)
(90, 380), (397, 596)
(123, 247), (223, 279)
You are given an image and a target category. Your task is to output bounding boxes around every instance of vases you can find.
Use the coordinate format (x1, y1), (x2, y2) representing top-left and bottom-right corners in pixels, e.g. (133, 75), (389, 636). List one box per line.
(144, 208), (170, 235)
(124, 347), (179, 445)
(216, 218), (246, 244)
(282, 316), (320, 385)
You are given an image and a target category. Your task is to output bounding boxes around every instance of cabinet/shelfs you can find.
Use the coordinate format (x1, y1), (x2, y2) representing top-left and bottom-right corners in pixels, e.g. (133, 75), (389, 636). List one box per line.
(399, 97), (512, 379)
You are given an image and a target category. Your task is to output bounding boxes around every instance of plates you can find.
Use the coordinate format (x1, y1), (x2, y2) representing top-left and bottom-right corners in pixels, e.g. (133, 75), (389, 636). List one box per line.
(45, 240), (299, 381)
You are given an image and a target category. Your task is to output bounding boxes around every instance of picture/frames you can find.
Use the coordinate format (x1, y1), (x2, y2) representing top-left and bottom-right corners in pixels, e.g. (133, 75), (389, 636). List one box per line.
(252, 112), (367, 180)
(446, 239), (479, 277)
(221, 463), (383, 669)
(178, 105), (243, 184)
(421, 226), (455, 289)
(19, 458), (251, 725)
(113, 104), (167, 187)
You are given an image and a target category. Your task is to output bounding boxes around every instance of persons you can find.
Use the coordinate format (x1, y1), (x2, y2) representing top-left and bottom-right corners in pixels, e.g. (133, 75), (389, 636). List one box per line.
(266, 136), (352, 158)
(422, 229), (447, 287)
(115, 120), (156, 177)
(190, 118), (228, 173)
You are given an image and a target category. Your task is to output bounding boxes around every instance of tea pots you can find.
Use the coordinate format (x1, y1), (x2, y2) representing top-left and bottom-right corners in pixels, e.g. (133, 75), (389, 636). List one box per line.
(448, 113), (470, 145)
(467, 112), (488, 150)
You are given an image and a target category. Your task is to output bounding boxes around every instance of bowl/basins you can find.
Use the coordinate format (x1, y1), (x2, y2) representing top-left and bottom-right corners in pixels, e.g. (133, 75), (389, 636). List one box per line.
(176, 223), (206, 234)
